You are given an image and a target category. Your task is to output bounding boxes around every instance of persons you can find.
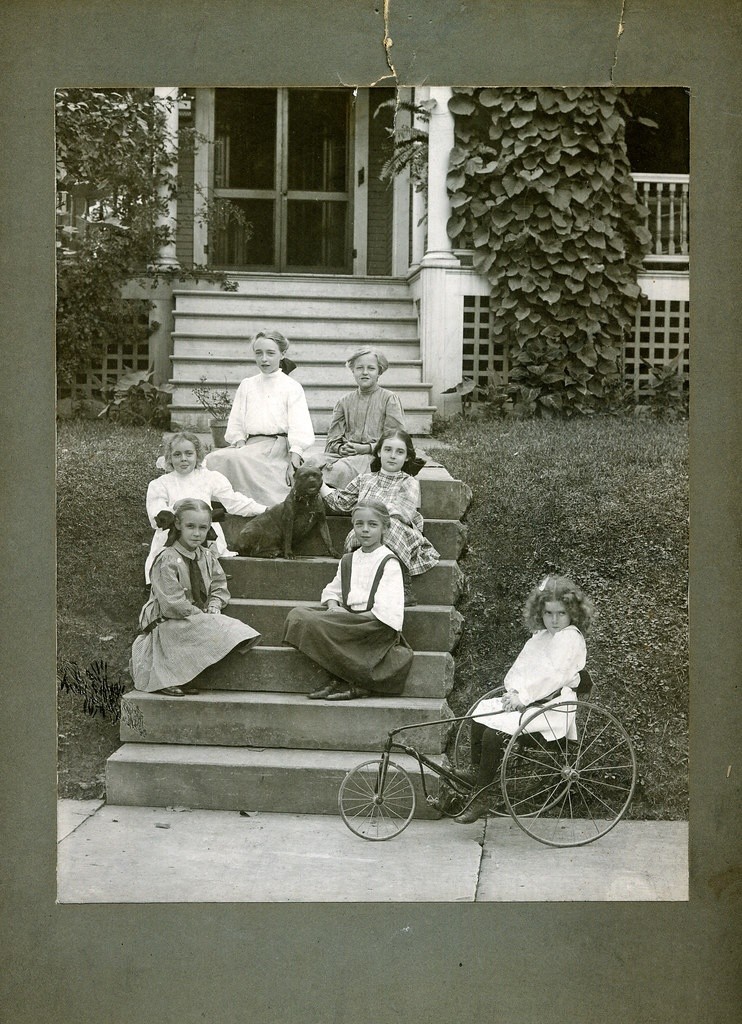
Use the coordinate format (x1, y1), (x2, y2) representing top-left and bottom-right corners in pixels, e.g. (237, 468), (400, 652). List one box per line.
(320, 347), (410, 486)
(282, 501), (413, 702)
(146, 434), (271, 584)
(202, 329), (316, 517)
(455, 575), (592, 823)
(320, 429), (441, 607)
(131, 499), (260, 695)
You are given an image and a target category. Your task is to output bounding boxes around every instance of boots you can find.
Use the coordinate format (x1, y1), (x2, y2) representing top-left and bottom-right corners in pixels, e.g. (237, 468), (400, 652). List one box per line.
(403, 572), (416, 606)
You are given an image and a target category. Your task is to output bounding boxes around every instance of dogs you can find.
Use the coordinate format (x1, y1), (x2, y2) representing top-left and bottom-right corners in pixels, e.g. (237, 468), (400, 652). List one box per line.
(227, 461), (341, 560)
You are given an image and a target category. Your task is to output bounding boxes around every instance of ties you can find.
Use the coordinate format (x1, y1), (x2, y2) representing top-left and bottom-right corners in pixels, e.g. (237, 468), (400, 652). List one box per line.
(188, 552), (208, 607)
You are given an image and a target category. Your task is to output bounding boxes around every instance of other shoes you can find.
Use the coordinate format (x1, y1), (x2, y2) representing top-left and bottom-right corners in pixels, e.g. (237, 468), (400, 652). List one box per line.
(224, 572), (231, 578)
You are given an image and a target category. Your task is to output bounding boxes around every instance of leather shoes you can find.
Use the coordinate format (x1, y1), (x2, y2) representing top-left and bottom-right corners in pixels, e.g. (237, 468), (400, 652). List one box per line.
(307, 676), (371, 701)
(454, 786), (491, 825)
(159, 683), (197, 696)
